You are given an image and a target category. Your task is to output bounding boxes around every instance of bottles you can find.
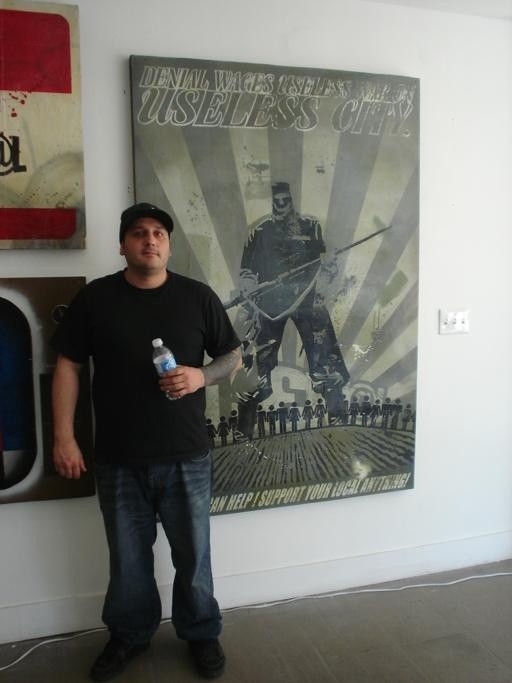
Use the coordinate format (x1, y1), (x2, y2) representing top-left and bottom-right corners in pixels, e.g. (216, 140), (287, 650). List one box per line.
(150, 338), (185, 401)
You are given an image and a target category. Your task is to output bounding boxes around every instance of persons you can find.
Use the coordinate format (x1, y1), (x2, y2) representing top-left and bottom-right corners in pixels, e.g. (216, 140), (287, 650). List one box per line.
(228, 181), (351, 440)
(48, 201), (245, 681)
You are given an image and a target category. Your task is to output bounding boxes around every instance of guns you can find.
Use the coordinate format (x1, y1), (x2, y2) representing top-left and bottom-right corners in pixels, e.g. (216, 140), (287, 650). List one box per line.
(220, 223), (397, 309)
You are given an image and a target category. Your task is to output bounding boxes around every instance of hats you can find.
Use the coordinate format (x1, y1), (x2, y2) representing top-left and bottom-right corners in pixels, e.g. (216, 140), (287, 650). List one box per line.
(122, 202), (174, 232)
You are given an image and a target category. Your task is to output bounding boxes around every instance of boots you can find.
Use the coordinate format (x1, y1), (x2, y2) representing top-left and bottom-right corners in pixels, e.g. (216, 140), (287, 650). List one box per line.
(323, 389), (349, 427)
(234, 404), (257, 445)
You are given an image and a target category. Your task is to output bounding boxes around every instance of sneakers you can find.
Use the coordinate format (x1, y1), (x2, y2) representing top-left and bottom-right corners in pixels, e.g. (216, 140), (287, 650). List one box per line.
(89, 636), (151, 683)
(188, 634), (226, 678)
